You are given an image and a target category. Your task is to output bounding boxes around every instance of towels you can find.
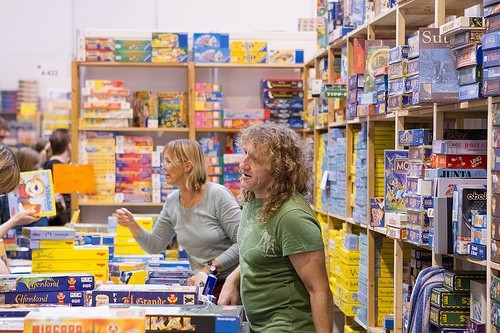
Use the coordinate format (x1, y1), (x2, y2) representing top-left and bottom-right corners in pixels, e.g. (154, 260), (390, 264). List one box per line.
(406, 266), (448, 333)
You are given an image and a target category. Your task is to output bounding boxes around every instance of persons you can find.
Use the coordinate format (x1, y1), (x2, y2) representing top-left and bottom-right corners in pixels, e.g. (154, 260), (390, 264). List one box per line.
(218, 120), (333, 333)
(0, 119), (73, 240)
(117, 139), (243, 305)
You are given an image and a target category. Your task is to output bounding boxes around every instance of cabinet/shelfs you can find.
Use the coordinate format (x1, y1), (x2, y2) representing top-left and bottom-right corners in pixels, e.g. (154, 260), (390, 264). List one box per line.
(304, 0), (500, 333)
(72, 60), (305, 223)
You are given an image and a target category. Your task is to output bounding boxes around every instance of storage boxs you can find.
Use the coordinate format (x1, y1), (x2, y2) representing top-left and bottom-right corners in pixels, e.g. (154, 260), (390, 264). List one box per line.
(0, 215), (250, 333)
(7, 169), (57, 218)
(303, 0), (500, 333)
(76, 28), (304, 203)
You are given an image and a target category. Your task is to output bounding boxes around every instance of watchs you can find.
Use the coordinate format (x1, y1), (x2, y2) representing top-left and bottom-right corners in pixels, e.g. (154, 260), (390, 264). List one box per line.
(207, 261), (218, 275)
